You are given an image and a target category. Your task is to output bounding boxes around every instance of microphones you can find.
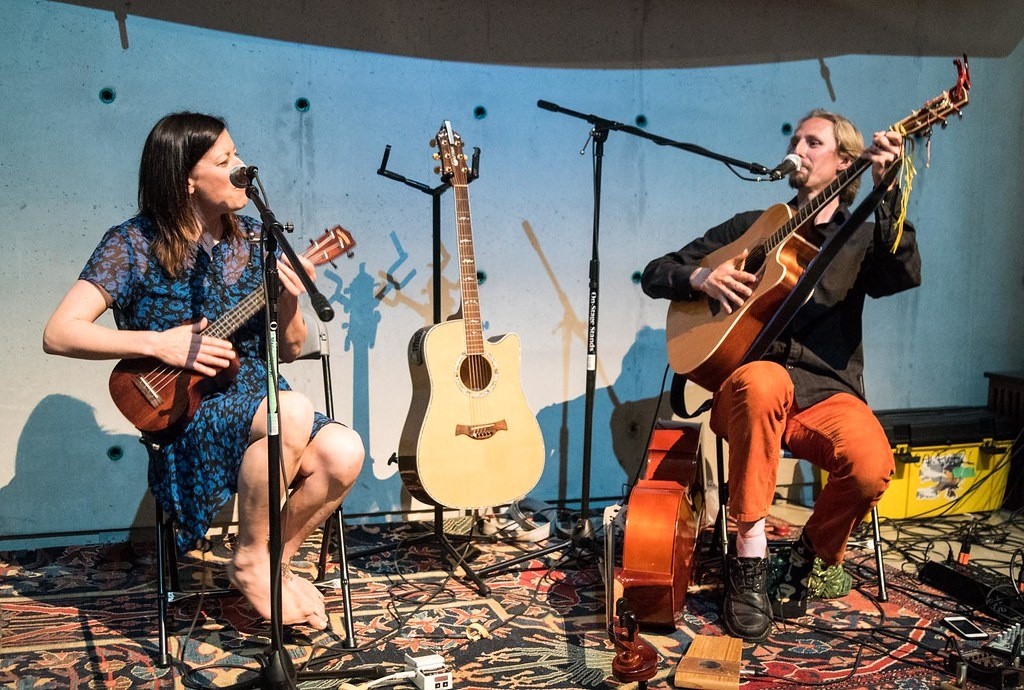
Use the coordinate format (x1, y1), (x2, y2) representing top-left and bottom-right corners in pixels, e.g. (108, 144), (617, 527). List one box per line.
(230, 165), (259, 189)
(768, 154), (802, 181)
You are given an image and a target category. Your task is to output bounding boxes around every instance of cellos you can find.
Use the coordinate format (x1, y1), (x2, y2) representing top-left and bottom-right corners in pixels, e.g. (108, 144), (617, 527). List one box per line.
(596, 425), (707, 685)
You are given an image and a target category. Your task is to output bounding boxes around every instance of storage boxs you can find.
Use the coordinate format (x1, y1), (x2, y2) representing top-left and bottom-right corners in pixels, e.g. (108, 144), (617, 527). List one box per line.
(820, 405), (1021, 523)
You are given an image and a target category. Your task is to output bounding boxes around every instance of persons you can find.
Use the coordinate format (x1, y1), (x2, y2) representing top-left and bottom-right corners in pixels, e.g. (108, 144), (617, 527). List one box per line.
(642, 110), (921, 642)
(42, 112), (364, 632)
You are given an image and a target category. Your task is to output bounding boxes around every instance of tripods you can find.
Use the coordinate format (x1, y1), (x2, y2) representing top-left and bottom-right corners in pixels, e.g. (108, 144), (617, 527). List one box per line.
(214, 100), (774, 690)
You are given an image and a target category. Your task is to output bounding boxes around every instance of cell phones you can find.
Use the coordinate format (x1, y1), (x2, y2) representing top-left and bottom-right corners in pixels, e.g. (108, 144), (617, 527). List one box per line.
(941, 616), (989, 641)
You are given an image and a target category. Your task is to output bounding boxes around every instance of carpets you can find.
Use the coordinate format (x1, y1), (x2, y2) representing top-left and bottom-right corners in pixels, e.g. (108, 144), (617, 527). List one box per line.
(0, 507), (1024, 690)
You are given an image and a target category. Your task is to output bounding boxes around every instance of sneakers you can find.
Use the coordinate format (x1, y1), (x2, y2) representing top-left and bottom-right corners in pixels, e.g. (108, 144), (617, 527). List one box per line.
(770, 545), (810, 618)
(727, 557), (774, 642)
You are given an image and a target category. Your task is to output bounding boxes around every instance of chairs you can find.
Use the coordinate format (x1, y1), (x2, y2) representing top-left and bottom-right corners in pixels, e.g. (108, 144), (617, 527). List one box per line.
(710, 376), (890, 604)
(155, 312), (356, 669)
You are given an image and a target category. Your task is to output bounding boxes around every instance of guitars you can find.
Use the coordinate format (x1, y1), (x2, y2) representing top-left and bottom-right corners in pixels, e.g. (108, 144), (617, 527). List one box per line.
(107, 222), (358, 443)
(664, 48), (976, 395)
(394, 118), (547, 514)
